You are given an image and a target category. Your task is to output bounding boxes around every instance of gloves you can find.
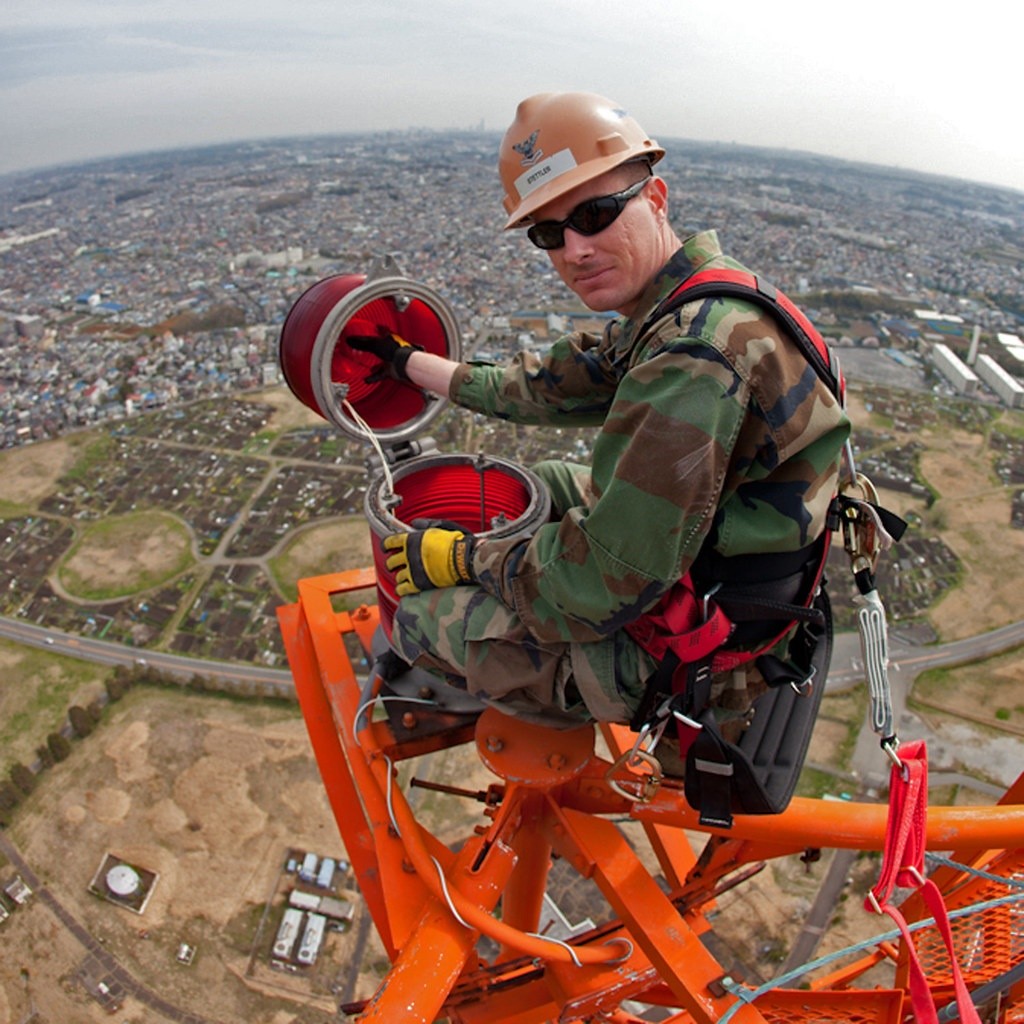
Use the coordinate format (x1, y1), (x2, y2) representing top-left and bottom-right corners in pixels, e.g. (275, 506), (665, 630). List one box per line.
(381, 519), (473, 596)
(345, 324), (415, 384)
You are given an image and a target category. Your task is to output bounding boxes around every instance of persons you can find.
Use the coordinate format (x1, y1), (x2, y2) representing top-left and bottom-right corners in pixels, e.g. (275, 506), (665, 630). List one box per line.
(346, 91), (853, 728)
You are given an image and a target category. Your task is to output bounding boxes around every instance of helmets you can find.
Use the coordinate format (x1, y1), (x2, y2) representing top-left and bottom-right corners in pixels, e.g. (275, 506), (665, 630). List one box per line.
(498, 93), (666, 230)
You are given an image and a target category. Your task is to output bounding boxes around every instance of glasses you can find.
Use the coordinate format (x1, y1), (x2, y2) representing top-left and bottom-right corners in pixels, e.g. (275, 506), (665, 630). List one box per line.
(528, 176), (654, 250)
